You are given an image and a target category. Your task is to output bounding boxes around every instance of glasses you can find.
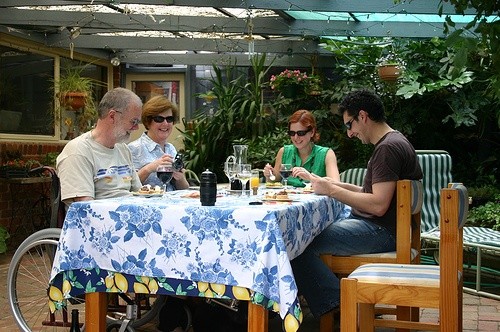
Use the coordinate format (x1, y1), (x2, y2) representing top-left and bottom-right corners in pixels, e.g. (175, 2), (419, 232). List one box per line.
(117, 110), (139, 126)
(287, 129), (311, 136)
(345, 112), (369, 130)
(152, 116), (174, 124)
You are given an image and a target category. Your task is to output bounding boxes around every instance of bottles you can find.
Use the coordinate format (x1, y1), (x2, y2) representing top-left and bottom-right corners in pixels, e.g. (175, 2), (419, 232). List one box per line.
(200, 169), (216, 206)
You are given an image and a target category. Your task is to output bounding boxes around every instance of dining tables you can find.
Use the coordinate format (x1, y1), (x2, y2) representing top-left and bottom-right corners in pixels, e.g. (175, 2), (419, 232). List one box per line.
(46, 184), (352, 332)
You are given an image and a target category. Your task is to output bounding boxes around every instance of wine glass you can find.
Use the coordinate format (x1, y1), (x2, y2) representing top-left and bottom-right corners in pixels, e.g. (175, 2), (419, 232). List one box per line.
(155, 164), (174, 198)
(225, 162), (259, 199)
(278, 164), (293, 190)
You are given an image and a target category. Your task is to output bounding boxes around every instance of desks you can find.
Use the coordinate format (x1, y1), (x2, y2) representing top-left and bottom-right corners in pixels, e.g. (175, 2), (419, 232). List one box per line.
(0, 177), (53, 257)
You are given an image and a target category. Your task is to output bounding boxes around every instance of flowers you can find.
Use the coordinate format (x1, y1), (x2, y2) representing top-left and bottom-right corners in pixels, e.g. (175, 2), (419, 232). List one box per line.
(269, 68), (307, 91)
(0, 159), (28, 168)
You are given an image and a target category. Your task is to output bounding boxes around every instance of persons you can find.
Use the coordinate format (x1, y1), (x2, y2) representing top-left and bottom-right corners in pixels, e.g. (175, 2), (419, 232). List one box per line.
(55, 88), (145, 201)
(291, 89), (424, 318)
(263, 110), (341, 186)
(127, 95), (190, 191)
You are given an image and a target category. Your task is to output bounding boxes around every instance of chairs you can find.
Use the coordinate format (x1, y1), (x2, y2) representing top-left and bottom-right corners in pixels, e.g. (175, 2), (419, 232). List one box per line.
(318, 149), (500, 332)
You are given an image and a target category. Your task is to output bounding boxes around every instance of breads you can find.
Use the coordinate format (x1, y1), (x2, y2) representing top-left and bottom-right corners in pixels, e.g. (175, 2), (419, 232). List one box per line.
(276, 194), (289, 199)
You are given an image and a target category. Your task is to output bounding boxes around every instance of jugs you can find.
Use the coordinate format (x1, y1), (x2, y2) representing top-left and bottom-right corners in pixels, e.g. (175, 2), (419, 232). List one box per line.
(225, 144), (250, 190)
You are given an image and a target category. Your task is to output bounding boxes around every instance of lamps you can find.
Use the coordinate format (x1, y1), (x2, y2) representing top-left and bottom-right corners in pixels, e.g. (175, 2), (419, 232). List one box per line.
(65, 25), (80, 39)
(110, 49), (122, 65)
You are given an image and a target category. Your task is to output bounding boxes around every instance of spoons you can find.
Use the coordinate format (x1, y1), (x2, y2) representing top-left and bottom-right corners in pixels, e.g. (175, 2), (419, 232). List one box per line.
(268, 163), (276, 181)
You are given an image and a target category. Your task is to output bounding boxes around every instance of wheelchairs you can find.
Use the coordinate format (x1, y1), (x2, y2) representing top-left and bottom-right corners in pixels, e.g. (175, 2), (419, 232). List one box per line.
(7, 165), (240, 332)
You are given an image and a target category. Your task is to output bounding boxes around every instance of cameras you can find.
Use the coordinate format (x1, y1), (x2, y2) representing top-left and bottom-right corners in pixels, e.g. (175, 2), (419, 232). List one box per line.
(172, 155), (184, 172)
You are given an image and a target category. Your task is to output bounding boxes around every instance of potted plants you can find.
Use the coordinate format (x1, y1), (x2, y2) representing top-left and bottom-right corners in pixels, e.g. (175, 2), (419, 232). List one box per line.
(371, 52), (407, 99)
(45, 58), (111, 140)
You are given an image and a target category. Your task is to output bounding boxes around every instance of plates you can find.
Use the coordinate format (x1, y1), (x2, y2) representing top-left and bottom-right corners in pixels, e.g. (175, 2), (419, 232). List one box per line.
(261, 185), (314, 202)
(131, 192), (163, 198)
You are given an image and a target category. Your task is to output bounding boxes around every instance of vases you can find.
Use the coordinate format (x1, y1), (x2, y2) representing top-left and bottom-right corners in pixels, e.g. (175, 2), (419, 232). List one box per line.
(283, 85), (295, 99)
(0, 167), (31, 178)
